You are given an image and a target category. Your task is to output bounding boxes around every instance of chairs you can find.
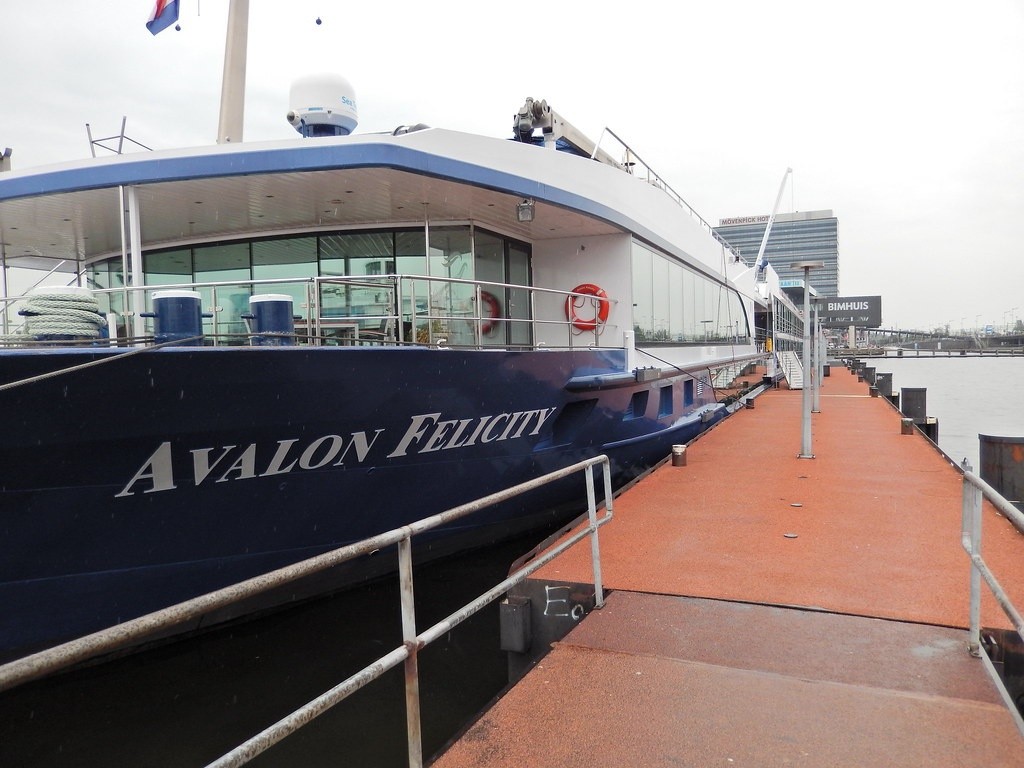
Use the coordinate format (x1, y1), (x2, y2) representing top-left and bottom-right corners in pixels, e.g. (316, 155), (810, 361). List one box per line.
(357, 313), (391, 346)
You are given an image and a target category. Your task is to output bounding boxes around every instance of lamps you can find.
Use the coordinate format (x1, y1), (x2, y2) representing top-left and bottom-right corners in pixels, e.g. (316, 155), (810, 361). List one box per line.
(516, 200), (536, 223)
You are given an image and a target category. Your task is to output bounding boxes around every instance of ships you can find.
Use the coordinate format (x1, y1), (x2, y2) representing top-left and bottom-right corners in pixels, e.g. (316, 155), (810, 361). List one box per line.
(0, 1), (814, 686)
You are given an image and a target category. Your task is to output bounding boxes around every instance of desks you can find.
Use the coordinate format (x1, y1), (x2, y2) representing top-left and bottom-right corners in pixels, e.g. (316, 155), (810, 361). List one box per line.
(295, 322), (360, 346)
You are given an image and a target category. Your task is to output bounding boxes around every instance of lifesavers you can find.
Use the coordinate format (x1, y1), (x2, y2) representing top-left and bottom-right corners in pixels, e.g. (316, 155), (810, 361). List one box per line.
(564, 283), (610, 331)
(463, 290), (500, 335)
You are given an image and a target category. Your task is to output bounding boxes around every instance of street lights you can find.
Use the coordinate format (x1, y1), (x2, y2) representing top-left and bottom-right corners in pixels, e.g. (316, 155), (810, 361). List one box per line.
(798, 260), (824, 458)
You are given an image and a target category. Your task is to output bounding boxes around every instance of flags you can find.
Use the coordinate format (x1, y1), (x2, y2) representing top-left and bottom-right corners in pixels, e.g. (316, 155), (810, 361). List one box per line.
(147, 0), (179, 36)
